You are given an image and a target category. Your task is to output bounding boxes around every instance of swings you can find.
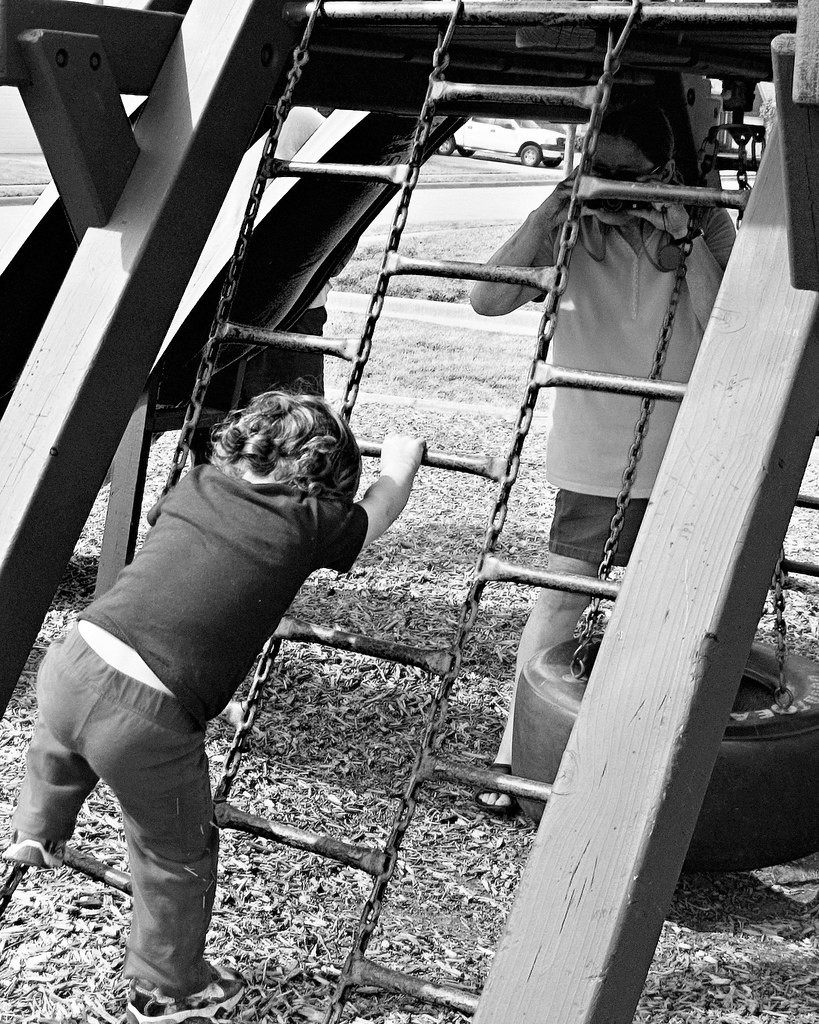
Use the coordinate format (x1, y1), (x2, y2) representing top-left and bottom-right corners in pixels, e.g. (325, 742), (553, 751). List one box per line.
(509, 125), (819, 873)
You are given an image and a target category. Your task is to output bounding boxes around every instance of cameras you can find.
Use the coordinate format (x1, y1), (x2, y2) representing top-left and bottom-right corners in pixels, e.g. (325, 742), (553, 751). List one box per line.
(586, 198), (652, 214)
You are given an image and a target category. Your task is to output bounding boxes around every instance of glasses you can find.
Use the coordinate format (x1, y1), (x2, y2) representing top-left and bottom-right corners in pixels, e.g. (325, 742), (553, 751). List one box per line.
(593, 164), (663, 180)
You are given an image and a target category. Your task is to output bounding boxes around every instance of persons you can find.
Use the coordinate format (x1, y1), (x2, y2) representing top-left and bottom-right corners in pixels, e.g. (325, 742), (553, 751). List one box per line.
(1, 390), (429, 1024)
(474, 106), (738, 817)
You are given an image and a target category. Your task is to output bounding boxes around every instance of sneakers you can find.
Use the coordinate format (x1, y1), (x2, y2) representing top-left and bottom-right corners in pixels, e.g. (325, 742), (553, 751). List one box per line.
(127, 967), (246, 1023)
(5, 831), (66, 867)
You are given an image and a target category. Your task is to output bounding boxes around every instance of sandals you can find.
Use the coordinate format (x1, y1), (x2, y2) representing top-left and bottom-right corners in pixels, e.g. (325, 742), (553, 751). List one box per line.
(473, 762), (515, 811)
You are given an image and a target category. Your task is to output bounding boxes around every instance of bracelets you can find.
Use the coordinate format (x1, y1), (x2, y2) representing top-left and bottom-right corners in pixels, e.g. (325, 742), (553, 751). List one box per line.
(672, 228), (705, 245)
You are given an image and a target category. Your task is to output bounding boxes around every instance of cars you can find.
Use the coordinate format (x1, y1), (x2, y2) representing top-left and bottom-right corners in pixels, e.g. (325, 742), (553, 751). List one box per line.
(433, 111), (568, 170)
